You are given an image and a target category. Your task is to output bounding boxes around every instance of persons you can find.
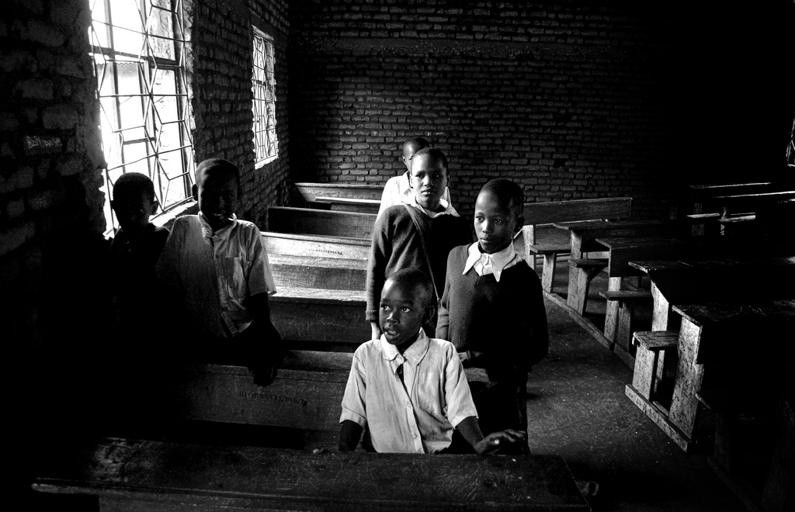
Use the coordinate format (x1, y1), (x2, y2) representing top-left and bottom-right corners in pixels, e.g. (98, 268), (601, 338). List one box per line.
(337, 265), (525, 457)
(166, 156), (279, 388)
(365, 146), (476, 342)
(98, 171), (207, 444)
(435, 179), (551, 455)
(376, 135), (452, 218)
